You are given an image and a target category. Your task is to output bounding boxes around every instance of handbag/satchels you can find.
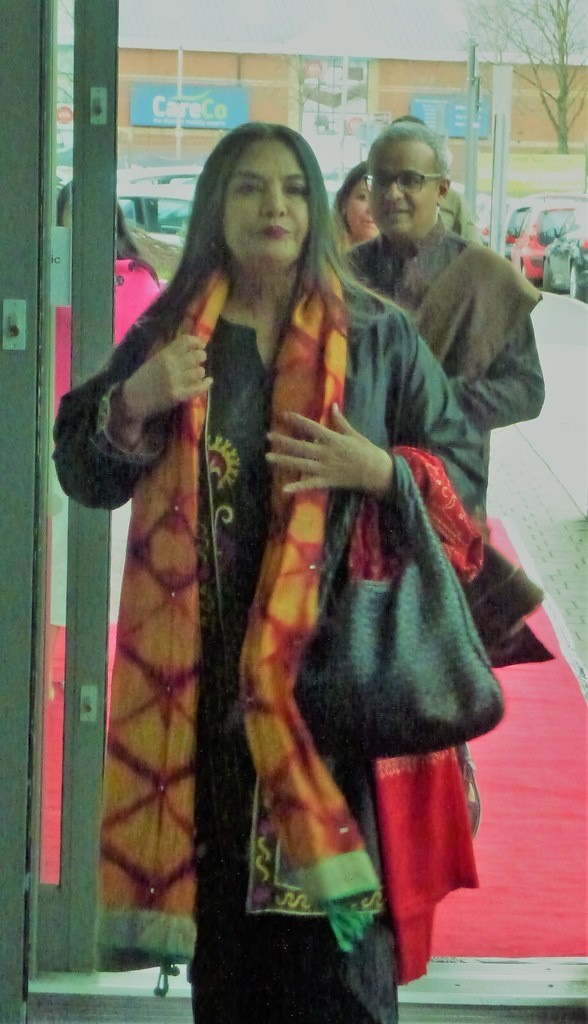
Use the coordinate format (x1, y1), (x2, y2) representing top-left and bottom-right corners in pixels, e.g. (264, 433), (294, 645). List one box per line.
(293, 452), (504, 757)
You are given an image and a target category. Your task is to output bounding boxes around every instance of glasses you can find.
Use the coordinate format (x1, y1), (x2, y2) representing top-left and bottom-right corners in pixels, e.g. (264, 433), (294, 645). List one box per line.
(363, 171), (442, 194)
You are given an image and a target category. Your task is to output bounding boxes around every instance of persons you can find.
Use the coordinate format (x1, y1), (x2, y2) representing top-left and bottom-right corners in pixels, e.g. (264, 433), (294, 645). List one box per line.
(53, 128), (557, 841)
(51, 123), (485, 1024)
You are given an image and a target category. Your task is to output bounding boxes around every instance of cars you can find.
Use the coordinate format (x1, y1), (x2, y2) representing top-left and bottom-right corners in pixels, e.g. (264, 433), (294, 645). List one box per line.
(512, 200), (579, 287)
(541, 201), (588, 300)
(502, 205), (534, 259)
(476, 199), (492, 245)
(53, 166), (205, 258)
